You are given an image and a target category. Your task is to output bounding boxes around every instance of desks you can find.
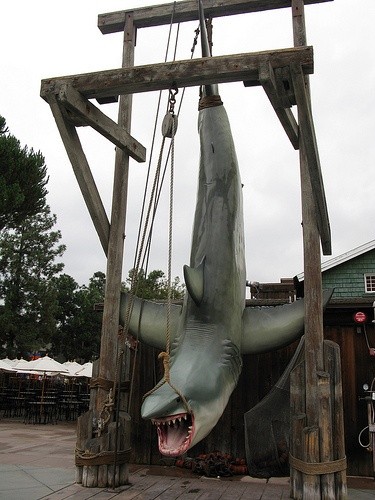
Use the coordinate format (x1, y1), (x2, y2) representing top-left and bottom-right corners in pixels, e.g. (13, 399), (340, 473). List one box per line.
(0, 382), (90, 425)
